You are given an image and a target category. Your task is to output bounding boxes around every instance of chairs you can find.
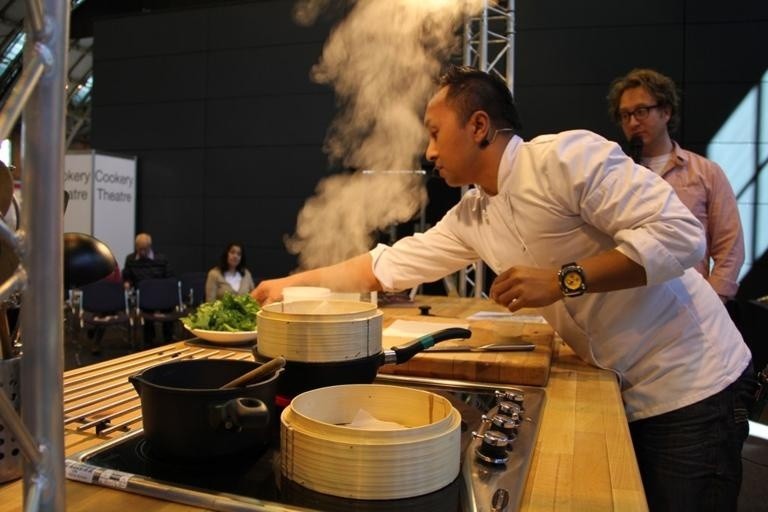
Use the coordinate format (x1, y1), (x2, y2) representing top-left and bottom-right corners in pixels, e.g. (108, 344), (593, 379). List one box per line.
(75, 271), (204, 365)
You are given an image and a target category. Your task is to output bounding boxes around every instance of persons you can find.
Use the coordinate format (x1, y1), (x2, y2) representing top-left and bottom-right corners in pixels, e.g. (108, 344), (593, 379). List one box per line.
(205, 238), (256, 306)
(604, 67), (746, 307)
(248, 62), (758, 512)
(121, 232), (179, 350)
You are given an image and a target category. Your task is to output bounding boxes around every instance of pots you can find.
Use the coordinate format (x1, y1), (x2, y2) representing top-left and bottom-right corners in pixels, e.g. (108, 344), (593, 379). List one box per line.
(126, 359), (284, 466)
(250, 326), (471, 429)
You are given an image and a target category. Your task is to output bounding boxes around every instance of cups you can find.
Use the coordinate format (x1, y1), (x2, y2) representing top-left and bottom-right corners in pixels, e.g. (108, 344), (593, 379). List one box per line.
(281, 287), (331, 303)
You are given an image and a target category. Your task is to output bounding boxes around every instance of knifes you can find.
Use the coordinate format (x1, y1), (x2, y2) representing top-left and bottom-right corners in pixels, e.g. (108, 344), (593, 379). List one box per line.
(422, 338), (537, 354)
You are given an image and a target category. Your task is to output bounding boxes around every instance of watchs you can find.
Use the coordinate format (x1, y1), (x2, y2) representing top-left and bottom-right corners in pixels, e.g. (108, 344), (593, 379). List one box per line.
(557, 262), (589, 298)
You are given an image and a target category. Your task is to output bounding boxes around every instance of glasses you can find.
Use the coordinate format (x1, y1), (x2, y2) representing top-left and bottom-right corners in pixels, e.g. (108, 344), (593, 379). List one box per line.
(619, 105), (658, 124)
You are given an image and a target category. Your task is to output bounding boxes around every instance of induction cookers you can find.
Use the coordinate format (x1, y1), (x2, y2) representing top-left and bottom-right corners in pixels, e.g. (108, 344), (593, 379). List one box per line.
(82, 370), (549, 511)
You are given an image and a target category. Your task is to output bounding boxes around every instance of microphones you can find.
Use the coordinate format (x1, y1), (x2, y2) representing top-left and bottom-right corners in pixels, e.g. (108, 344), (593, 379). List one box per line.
(631, 135), (643, 163)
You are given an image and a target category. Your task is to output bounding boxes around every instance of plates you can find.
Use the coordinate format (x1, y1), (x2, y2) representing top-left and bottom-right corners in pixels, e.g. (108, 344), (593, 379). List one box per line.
(183, 323), (258, 343)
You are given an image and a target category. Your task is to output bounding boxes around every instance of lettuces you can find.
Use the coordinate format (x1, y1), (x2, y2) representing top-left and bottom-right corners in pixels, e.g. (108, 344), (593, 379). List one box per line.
(178, 294), (260, 330)
